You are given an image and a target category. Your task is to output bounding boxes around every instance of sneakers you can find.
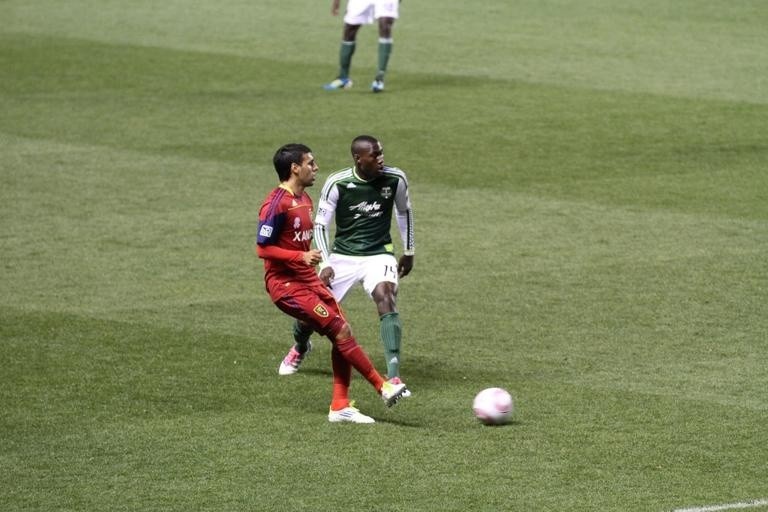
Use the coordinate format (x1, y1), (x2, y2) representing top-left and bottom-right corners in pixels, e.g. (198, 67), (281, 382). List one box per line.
(277, 340), (313, 378)
(370, 79), (385, 92)
(319, 78), (352, 91)
(327, 403), (377, 425)
(381, 375), (412, 409)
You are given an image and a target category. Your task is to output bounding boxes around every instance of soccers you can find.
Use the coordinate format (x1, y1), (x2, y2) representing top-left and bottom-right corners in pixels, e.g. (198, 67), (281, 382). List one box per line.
(471, 386), (513, 425)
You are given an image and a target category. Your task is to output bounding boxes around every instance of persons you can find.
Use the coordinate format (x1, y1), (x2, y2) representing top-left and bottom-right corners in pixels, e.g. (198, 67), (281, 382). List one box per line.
(255, 142), (408, 424)
(322, 1), (403, 94)
(275, 135), (414, 399)
